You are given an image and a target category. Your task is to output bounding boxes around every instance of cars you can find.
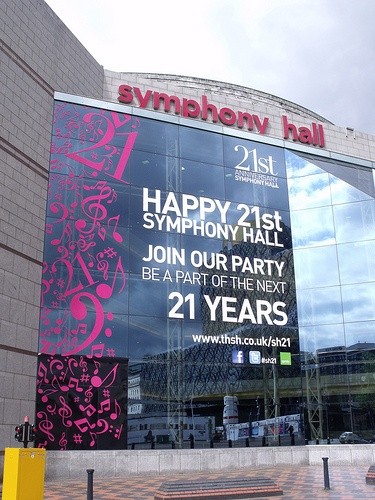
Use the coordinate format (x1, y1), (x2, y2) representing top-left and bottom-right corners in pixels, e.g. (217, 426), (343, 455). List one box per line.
(339, 432), (361, 445)
